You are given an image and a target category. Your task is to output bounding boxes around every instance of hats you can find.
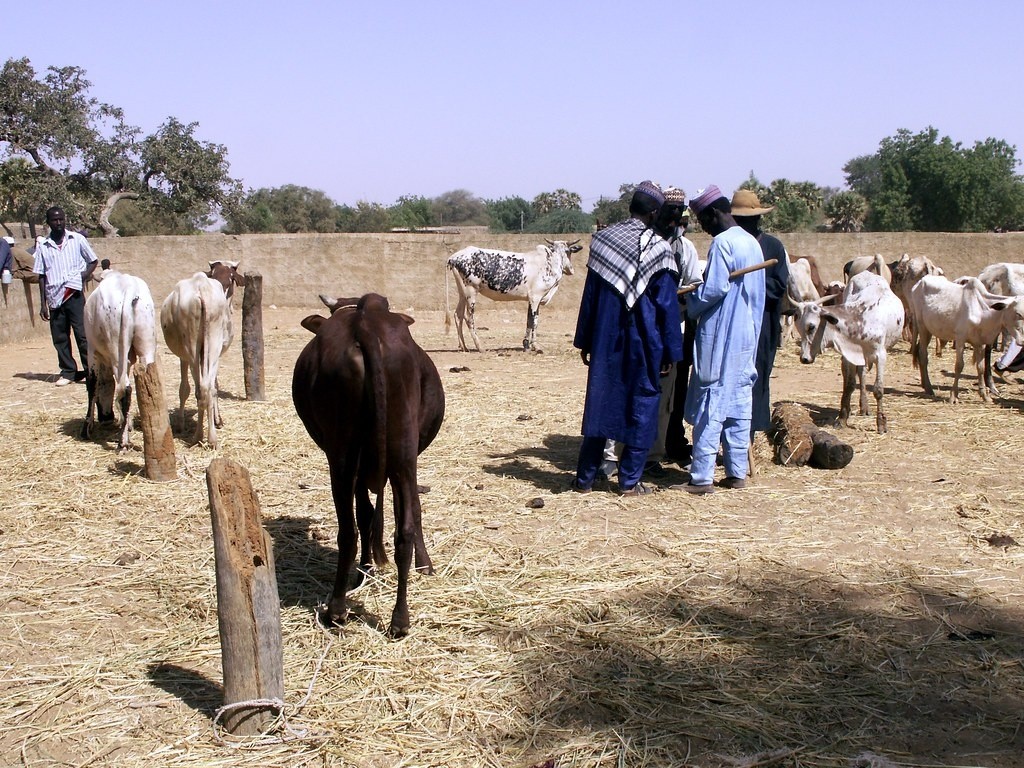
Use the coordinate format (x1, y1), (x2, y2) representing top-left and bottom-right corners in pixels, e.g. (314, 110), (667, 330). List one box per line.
(636, 179), (665, 206)
(688, 184), (723, 216)
(731, 189), (774, 215)
(661, 187), (685, 206)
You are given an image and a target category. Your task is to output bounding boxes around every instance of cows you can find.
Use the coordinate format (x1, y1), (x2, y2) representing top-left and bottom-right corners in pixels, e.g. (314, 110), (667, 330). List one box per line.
(887, 253), (943, 358)
(978, 263), (1024, 296)
(786, 270), (904, 437)
(843, 254), (892, 289)
(160, 260), (245, 449)
(443, 238), (583, 353)
(779, 254), (844, 346)
(910, 273), (1024, 407)
(292, 292), (445, 637)
(81, 269), (157, 448)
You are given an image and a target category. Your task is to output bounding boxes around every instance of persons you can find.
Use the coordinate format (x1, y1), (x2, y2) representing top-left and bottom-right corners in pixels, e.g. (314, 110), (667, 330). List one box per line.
(594, 188), (702, 479)
(93, 259), (113, 282)
(991, 336), (1024, 385)
(569, 180), (682, 497)
(33, 206), (99, 386)
(668, 185), (766, 495)
(0, 236), (45, 284)
(730, 190), (789, 448)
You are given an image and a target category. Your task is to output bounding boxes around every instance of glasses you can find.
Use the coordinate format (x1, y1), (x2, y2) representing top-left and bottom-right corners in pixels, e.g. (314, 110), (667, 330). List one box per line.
(678, 221), (688, 228)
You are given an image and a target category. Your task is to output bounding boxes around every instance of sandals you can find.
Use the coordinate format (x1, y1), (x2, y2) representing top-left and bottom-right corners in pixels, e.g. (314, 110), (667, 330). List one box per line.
(619, 483), (653, 496)
(571, 478), (592, 493)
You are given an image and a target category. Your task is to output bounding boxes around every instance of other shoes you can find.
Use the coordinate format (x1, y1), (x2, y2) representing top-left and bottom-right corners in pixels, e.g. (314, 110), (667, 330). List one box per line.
(56, 377), (71, 386)
(648, 462), (669, 477)
(669, 478), (715, 492)
(718, 477), (745, 488)
